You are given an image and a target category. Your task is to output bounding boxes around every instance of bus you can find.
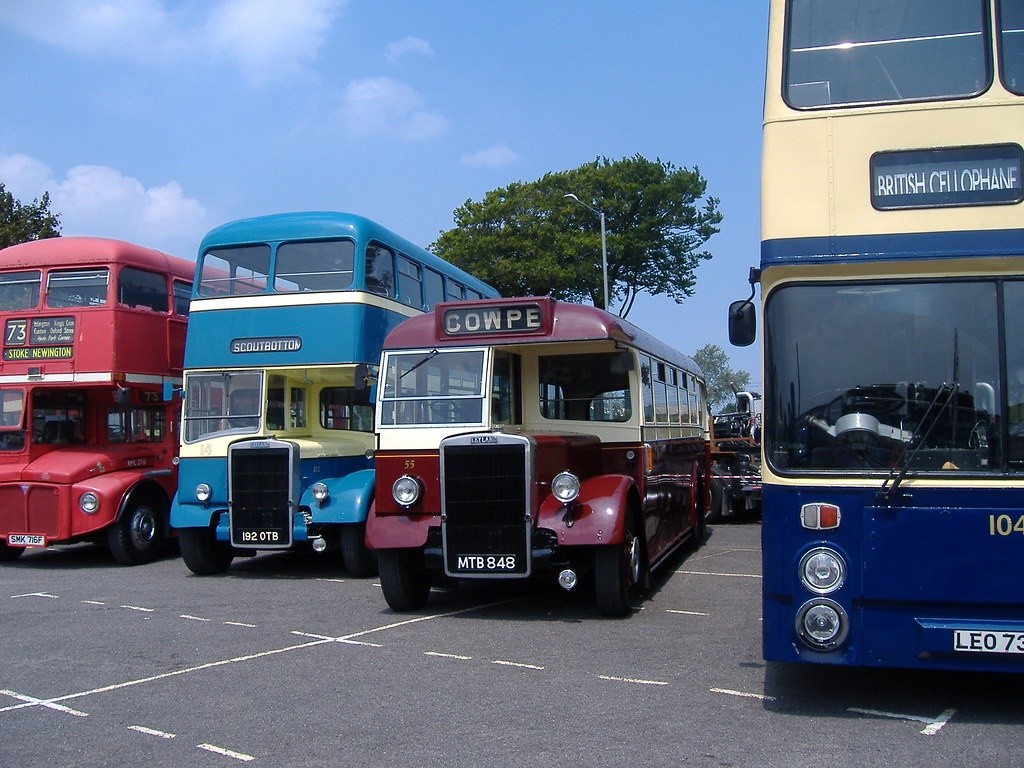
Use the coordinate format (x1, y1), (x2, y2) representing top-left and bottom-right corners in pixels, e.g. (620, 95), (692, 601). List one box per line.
(367, 297), (713, 620)
(726, 0), (1022, 677)
(0, 237), (292, 569)
(164, 209), (503, 577)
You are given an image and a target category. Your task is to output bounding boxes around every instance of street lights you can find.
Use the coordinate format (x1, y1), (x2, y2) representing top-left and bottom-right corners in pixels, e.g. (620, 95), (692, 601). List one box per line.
(564, 192), (609, 315)
